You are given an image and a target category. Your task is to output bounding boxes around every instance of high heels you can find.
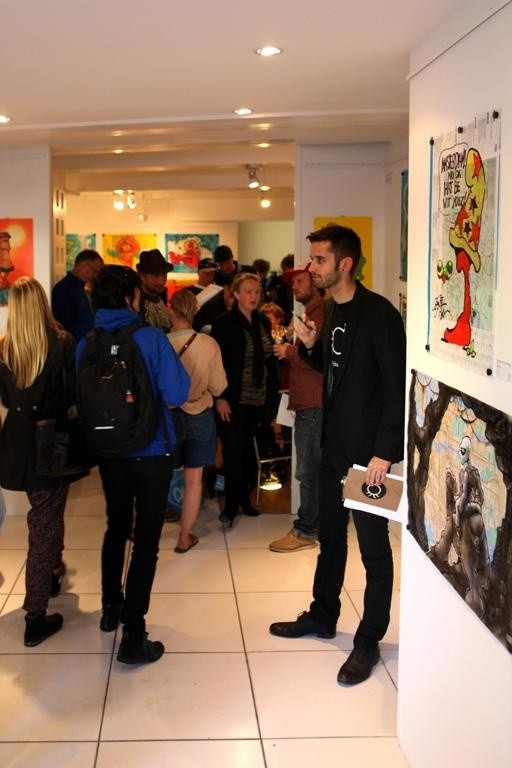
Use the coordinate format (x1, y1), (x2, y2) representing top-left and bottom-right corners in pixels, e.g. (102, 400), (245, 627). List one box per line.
(238, 499), (260, 517)
(219, 508), (236, 528)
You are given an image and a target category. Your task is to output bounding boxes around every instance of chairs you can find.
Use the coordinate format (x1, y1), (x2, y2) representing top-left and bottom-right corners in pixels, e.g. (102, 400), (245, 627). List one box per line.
(251, 436), (292, 507)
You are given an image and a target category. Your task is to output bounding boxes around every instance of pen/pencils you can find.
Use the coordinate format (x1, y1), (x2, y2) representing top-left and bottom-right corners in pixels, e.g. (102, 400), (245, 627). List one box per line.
(290, 309), (319, 337)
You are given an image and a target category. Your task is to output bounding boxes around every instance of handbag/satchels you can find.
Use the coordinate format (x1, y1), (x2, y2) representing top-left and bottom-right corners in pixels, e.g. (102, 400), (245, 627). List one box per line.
(0, 396), (91, 490)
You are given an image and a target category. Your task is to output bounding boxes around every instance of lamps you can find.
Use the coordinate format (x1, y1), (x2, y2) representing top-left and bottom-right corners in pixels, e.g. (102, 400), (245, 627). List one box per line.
(244, 163), (263, 189)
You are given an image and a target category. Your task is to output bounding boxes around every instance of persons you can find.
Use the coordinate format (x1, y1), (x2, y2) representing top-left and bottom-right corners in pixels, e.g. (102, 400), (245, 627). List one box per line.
(69, 263), (191, 665)
(1, 276), (94, 648)
(269, 222), (405, 686)
(269, 260), (330, 553)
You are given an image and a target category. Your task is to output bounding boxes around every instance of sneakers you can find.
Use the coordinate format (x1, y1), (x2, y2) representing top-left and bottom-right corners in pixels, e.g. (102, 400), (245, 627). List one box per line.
(98, 594), (124, 631)
(163, 505), (180, 524)
(23, 610), (65, 647)
(116, 618), (165, 665)
(48, 557), (65, 596)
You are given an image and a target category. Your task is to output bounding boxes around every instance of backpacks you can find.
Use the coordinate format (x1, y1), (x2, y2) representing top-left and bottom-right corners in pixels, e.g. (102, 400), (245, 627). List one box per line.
(80, 322), (157, 459)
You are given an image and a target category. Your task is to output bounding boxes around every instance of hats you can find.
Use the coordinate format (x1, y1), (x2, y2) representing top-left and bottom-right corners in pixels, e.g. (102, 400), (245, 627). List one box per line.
(135, 248), (174, 274)
(282, 262), (315, 283)
(198, 257), (220, 272)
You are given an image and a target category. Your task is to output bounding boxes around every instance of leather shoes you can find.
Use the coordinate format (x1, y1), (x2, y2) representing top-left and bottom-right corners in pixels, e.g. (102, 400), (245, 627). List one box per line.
(269, 610), (337, 639)
(269, 531), (318, 552)
(336, 642), (380, 684)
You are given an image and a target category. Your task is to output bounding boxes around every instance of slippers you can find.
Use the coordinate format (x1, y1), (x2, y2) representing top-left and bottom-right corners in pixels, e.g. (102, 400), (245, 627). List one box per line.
(174, 534), (199, 553)
